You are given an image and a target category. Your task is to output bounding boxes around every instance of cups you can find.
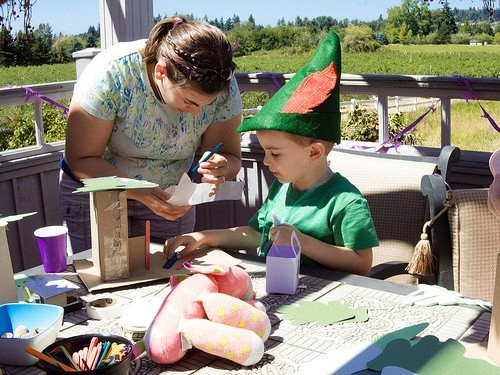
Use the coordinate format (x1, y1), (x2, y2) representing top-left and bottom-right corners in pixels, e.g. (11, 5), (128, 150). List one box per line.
(34, 225), (68, 273)
(34, 333), (134, 375)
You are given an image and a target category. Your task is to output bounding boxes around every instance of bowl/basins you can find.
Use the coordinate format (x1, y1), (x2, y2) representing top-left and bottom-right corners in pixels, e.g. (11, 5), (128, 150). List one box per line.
(0, 303), (64, 366)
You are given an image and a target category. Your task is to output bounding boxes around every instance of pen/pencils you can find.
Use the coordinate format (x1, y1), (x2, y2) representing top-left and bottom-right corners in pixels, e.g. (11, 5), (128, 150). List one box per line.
(60, 345), (81, 371)
(191, 142), (224, 173)
(24, 347), (75, 372)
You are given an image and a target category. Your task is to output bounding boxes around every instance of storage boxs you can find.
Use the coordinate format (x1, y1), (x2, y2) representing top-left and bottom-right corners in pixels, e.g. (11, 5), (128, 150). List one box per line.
(86, 190), (131, 283)
(264, 229), (303, 295)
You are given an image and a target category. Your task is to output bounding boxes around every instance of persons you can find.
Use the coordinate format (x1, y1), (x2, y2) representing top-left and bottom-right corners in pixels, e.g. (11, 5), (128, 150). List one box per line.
(59, 16), (243, 255)
(162, 30), (379, 276)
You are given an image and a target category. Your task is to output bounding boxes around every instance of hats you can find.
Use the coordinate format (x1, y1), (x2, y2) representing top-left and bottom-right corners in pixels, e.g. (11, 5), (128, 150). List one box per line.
(236, 30), (341, 145)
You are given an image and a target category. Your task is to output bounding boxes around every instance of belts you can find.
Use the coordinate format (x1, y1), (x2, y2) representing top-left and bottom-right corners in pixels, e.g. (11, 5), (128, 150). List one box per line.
(59, 153), (85, 186)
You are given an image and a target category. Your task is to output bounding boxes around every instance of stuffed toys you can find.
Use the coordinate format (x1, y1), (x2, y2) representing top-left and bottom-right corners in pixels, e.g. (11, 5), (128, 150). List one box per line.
(130, 262), (271, 366)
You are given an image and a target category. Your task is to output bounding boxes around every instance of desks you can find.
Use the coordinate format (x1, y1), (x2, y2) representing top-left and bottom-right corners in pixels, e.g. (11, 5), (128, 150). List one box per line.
(0, 238), (500, 375)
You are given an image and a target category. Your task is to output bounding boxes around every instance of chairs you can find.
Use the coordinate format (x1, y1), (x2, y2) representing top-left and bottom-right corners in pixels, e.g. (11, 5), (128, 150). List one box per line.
(330, 141), (500, 304)
